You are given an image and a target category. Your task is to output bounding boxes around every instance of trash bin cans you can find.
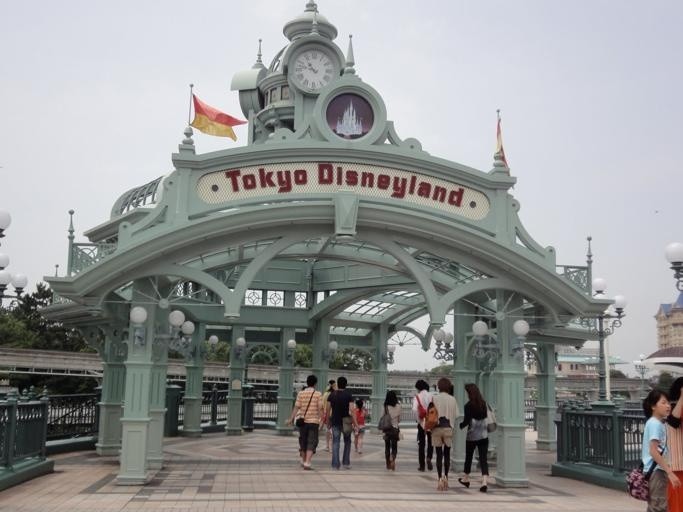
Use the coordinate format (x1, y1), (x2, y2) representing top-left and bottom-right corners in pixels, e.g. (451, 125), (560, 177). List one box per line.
(242, 384), (256, 432)
(553, 420), (589, 464)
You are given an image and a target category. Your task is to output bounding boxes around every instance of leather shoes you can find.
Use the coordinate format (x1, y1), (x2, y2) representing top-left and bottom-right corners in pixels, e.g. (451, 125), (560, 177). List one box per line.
(479, 484), (487, 493)
(418, 465), (426, 472)
(458, 477), (471, 488)
(426, 458), (433, 472)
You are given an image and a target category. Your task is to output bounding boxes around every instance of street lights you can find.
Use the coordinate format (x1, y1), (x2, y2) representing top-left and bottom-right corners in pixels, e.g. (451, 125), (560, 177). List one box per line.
(0, 210), (26, 315)
(632, 354), (650, 400)
(577, 275), (628, 411)
(665, 239), (683, 295)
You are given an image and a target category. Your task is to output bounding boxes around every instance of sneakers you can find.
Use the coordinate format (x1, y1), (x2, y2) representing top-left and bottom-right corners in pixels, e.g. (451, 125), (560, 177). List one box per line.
(331, 462), (352, 470)
(386, 460), (396, 470)
(299, 459), (313, 470)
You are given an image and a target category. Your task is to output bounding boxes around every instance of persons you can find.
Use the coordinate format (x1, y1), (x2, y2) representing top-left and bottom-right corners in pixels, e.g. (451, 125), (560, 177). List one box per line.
(661, 376), (683, 511)
(458, 383), (498, 493)
(321, 379), (336, 454)
(641, 388), (681, 512)
(294, 385), (307, 457)
(410, 378), (434, 472)
(376, 390), (401, 470)
(353, 399), (366, 454)
(284, 374), (324, 469)
(429, 377), (460, 492)
(325, 376), (356, 473)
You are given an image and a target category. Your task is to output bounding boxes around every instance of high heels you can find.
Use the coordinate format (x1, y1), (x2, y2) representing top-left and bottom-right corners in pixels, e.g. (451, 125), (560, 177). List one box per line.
(437, 477), (449, 492)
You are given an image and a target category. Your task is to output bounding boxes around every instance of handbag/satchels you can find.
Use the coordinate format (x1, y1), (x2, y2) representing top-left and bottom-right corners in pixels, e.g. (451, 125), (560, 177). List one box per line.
(626, 426), (668, 501)
(486, 401), (497, 434)
(343, 416), (353, 434)
(425, 396), (438, 431)
(378, 405), (392, 432)
(416, 395), (427, 429)
(294, 417), (305, 427)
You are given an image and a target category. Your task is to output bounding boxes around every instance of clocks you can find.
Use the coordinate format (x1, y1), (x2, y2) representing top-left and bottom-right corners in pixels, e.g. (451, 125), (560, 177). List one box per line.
(288, 42), (340, 97)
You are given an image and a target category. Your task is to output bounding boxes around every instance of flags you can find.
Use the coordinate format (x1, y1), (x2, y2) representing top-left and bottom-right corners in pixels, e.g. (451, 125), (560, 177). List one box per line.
(189, 95), (247, 144)
(494, 116), (508, 169)
